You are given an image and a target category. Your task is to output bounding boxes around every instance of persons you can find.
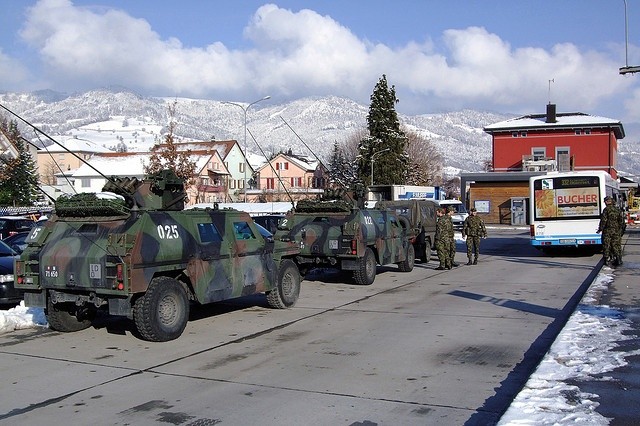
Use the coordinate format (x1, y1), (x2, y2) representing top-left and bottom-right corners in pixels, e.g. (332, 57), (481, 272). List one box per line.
(446, 206), (459, 267)
(461, 207), (487, 265)
(611, 196), (626, 264)
(435, 208), (451, 270)
(597, 196), (621, 268)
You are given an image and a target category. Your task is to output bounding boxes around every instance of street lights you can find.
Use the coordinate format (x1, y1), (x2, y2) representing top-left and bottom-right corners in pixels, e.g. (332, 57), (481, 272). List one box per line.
(371, 148), (390, 185)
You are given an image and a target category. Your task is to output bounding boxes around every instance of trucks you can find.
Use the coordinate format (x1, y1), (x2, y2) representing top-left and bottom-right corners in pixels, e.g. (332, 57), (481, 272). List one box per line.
(365, 184), (447, 210)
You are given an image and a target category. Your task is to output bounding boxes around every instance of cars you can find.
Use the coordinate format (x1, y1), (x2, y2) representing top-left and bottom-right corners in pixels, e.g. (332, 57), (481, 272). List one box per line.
(0, 216), (34, 239)
(0, 231), (29, 303)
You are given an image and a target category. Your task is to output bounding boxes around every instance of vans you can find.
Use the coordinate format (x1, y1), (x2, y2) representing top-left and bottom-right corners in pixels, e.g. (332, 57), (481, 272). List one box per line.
(429, 199), (469, 228)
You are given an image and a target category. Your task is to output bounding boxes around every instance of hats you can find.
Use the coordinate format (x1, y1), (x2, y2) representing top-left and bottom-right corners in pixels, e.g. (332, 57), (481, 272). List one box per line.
(471, 208), (476, 212)
(447, 206), (455, 211)
(604, 196), (610, 202)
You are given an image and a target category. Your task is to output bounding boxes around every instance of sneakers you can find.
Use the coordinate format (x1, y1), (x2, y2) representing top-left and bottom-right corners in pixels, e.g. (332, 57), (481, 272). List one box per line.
(619, 258), (623, 264)
(435, 267), (445, 270)
(467, 260), (472, 265)
(473, 260), (477, 265)
(614, 256), (620, 265)
(451, 261), (459, 267)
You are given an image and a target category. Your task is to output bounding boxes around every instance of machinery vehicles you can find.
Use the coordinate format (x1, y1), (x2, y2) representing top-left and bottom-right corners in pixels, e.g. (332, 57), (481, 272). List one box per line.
(0, 102), (302, 342)
(247, 116), (416, 285)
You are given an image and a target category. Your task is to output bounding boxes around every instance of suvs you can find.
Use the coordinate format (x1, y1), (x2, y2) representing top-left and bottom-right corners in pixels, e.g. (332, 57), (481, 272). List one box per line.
(374, 201), (439, 262)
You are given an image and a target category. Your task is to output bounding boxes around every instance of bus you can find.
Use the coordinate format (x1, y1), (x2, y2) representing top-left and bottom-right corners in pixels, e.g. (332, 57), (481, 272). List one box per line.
(529, 170), (620, 251)
